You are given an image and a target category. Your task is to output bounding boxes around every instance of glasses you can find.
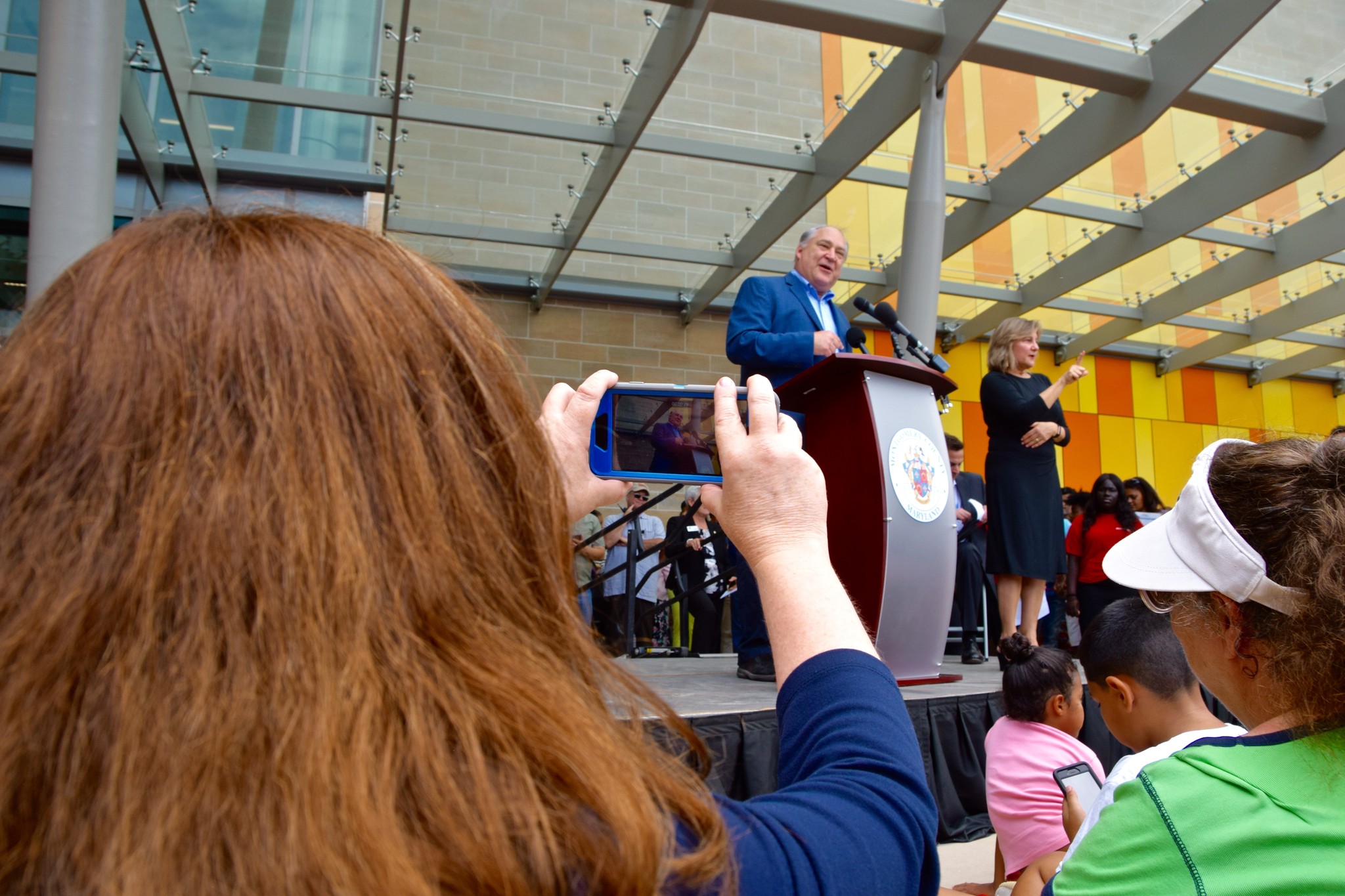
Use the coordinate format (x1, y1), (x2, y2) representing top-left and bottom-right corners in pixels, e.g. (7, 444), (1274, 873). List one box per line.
(634, 493), (648, 501)
(1125, 479), (1139, 485)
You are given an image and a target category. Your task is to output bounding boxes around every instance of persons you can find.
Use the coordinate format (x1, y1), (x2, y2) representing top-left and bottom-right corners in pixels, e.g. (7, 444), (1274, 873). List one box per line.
(953, 631), (1106, 896)
(1041, 430), (1345, 896)
(649, 409), (685, 473)
(0, 202), (942, 896)
(571, 434), (1166, 664)
(979, 318), (1088, 671)
(1009, 597), (1250, 895)
(725, 224), (852, 681)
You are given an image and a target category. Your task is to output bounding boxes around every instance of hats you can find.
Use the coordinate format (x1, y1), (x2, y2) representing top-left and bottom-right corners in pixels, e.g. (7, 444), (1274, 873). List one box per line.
(626, 482), (650, 496)
(1102, 439), (1309, 615)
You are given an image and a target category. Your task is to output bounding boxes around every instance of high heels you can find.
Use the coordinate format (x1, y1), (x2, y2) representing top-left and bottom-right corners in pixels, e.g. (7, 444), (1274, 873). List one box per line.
(996, 651), (1010, 670)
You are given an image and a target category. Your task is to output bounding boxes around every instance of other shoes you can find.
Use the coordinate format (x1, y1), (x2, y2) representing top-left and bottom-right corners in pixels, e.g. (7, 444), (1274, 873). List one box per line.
(736, 654), (777, 682)
(962, 639), (984, 664)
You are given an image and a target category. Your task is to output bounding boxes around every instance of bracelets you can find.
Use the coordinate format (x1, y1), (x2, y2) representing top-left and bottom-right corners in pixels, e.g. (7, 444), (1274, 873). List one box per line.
(1052, 424), (1061, 438)
